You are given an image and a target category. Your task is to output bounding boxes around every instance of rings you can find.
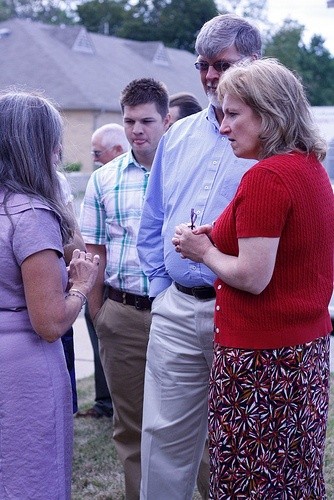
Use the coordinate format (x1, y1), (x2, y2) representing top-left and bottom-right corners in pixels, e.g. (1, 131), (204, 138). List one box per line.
(86, 257), (92, 261)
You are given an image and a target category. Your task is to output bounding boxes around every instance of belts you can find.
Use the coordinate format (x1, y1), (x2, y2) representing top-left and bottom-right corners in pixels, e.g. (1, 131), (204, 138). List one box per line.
(174, 282), (217, 299)
(105, 285), (155, 311)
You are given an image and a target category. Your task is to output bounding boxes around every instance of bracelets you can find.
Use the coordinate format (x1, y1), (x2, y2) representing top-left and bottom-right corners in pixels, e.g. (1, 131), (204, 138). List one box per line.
(65, 293), (83, 312)
(69, 289), (87, 305)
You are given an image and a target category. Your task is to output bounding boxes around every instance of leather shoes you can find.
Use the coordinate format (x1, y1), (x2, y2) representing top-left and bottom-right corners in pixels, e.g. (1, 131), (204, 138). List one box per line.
(78, 408), (102, 418)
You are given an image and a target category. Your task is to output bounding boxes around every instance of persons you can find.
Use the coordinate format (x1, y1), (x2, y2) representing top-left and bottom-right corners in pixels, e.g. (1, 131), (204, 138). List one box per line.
(170, 57), (334, 500)
(77, 77), (172, 500)
(0, 93), (100, 498)
(167, 94), (202, 129)
(90, 123), (132, 169)
(52, 140), (85, 415)
(140, 14), (262, 500)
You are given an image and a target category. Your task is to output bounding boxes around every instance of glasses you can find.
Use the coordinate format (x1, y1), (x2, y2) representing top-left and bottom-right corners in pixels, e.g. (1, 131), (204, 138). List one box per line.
(195, 59), (238, 72)
(90, 145), (114, 157)
